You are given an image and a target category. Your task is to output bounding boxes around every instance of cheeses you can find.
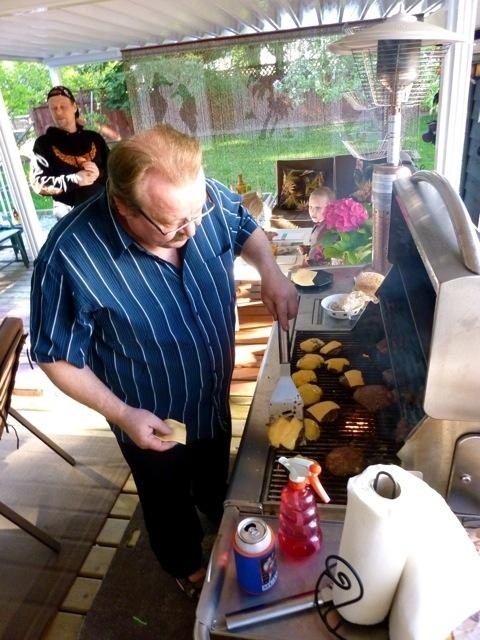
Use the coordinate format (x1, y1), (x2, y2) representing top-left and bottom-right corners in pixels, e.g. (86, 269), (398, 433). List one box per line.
(306, 399), (340, 422)
(325, 358), (349, 372)
(291, 370), (317, 385)
(297, 385), (322, 405)
(300, 338), (326, 353)
(269, 416), (293, 447)
(297, 354), (324, 369)
(280, 418), (304, 448)
(344, 368), (365, 387)
(304, 418), (320, 441)
(319, 339), (342, 354)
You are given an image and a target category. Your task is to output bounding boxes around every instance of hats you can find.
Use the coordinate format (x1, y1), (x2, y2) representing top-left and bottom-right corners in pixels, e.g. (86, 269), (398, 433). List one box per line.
(47, 85), (80, 118)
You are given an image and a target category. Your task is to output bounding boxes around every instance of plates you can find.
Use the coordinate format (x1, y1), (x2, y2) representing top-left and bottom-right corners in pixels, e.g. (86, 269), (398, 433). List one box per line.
(292, 267), (334, 290)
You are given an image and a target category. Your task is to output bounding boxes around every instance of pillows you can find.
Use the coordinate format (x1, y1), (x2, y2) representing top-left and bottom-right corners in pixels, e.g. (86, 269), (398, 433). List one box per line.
(279, 168), (328, 211)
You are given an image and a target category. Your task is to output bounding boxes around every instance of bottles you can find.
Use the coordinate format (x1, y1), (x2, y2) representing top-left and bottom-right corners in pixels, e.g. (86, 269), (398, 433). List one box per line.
(231, 517), (279, 596)
(236, 174), (247, 194)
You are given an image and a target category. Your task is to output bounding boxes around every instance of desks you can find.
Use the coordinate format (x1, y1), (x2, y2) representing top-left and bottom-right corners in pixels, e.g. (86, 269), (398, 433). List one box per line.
(0, 227), (30, 268)
(232, 228), (313, 331)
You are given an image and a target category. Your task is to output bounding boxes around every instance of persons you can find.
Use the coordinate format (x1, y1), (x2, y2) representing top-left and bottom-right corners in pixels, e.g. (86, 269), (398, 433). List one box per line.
(29, 126), (300, 606)
(310, 186), (334, 241)
(30, 86), (110, 221)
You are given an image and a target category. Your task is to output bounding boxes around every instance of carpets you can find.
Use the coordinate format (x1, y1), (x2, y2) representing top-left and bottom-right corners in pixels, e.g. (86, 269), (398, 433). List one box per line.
(77, 454), (235, 640)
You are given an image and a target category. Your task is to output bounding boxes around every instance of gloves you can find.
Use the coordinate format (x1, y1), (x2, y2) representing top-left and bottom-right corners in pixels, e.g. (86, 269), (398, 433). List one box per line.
(82, 160), (101, 180)
(77, 171), (95, 186)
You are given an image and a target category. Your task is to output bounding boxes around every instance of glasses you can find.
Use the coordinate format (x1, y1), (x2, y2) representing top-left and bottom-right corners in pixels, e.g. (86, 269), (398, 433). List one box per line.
(134, 185), (216, 238)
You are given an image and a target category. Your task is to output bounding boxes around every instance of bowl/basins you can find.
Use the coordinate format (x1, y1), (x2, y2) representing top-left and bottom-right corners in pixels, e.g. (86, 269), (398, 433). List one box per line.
(320, 294), (364, 319)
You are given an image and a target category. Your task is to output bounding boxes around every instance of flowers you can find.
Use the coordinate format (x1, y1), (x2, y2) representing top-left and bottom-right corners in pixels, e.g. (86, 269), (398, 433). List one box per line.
(309, 197), (372, 266)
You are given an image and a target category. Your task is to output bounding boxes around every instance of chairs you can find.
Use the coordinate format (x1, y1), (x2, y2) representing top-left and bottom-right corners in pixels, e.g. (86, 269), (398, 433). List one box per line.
(269, 155), (334, 229)
(335, 151), (388, 199)
(238, 191), (265, 228)
(0, 316), (76, 555)
